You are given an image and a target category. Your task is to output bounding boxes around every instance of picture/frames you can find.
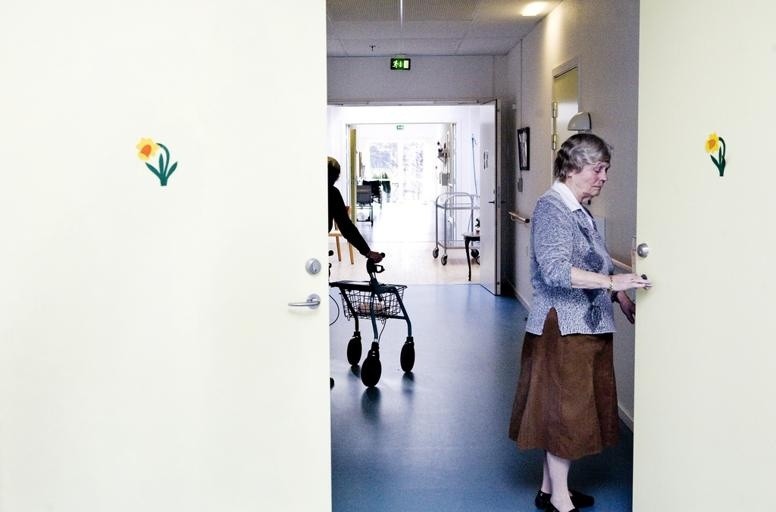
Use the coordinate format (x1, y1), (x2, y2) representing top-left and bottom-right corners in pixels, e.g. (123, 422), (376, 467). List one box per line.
(517, 127), (530, 171)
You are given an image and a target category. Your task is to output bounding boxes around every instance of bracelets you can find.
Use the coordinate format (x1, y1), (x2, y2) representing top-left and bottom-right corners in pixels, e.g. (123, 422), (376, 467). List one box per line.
(607, 275), (613, 292)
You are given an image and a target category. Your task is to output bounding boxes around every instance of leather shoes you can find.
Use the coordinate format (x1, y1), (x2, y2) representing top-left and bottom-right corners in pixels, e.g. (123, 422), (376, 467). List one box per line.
(535, 489), (595, 512)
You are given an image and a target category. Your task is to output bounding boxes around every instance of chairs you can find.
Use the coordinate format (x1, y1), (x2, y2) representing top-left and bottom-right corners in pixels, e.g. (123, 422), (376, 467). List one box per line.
(328, 206), (354, 265)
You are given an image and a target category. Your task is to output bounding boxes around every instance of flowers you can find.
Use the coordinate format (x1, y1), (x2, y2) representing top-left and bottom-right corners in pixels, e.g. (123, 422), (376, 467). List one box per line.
(136, 138), (178, 187)
(705, 132), (726, 176)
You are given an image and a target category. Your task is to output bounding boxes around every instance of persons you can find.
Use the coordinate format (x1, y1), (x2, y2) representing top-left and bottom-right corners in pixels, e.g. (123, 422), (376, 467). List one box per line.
(328, 156), (382, 390)
(510, 133), (652, 512)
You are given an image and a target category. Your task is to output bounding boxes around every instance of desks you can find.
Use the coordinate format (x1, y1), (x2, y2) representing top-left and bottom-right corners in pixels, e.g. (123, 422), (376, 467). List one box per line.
(462, 231), (480, 281)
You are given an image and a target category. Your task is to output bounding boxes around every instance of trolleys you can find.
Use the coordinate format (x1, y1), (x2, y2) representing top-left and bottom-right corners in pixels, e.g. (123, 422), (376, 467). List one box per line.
(328, 250), (415, 387)
(433, 192), (479, 265)
